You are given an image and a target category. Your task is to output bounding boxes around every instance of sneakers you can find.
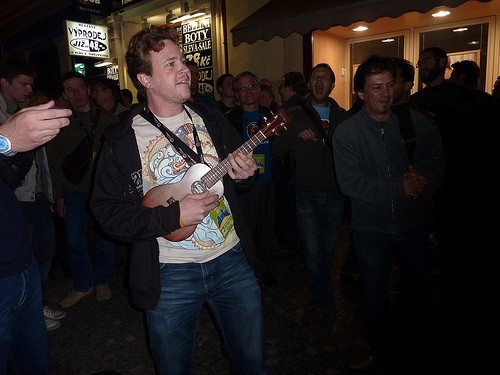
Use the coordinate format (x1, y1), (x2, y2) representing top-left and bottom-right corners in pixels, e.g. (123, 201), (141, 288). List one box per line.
(96, 284), (111, 303)
(43, 306), (66, 331)
(59, 286), (94, 308)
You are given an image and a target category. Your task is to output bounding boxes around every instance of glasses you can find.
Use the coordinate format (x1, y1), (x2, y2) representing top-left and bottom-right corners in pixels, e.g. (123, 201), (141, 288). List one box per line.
(236, 82), (257, 93)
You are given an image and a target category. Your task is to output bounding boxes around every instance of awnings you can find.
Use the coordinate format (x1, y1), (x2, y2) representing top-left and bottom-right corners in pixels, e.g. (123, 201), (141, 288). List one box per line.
(231, 1), (491, 46)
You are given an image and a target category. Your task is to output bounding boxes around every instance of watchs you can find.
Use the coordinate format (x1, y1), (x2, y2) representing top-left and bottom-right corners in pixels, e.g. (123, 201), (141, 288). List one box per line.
(0, 135), (17, 157)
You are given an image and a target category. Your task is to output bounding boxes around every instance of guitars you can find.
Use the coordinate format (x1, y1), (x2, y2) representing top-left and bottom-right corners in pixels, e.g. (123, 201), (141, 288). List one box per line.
(142, 107), (293, 243)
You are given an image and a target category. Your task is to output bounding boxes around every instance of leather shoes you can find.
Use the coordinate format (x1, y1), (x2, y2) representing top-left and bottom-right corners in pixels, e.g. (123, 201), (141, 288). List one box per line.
(301, 308), (323, 322)
(347, 350), (378, 369)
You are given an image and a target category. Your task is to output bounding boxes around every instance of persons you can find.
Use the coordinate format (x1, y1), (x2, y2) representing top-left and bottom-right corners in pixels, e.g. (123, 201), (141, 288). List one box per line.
(0, 23), (499, 375)
